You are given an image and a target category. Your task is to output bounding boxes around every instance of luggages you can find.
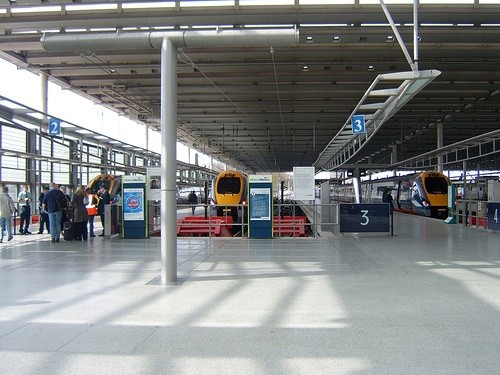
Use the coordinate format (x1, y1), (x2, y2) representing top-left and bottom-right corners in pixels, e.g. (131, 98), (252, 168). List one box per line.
(64, 220), (73, 241)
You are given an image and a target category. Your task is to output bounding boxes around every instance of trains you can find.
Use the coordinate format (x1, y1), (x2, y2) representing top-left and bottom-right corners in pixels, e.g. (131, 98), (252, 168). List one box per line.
(209, 170), (248, 220)
(315, 171), (454, 217)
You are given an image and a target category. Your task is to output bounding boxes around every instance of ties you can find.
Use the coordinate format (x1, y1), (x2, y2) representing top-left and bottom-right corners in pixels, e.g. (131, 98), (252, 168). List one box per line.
(25, 192), (29, 205)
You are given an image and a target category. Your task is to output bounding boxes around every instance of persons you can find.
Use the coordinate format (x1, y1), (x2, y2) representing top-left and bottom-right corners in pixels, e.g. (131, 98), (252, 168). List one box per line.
(97, 185), (110, 236)
(0, 186), (15, 243)
(150, 179), (158, 189)
(189, 191), (197, 215)
(18, 184), (33, 234)
(37, 182), (97, 243)
(383, 189), (394, 215)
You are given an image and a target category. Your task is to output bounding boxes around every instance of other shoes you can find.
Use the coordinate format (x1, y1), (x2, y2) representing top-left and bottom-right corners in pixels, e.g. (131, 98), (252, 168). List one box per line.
(52, 238), (59, 243)
(19, 229), (31, 234)
(98, 234), (104, 236)
(90, 235), (96, 237)
(37, 231), (43, 234)
(8, 236), (13, 241)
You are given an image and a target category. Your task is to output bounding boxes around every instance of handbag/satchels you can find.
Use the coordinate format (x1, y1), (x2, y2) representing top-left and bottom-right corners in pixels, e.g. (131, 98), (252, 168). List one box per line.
(87, 207), (98, 215)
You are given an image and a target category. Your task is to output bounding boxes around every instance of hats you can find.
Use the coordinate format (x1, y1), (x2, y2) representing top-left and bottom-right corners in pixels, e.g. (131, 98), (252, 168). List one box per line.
(43, 186), (50, 190)
